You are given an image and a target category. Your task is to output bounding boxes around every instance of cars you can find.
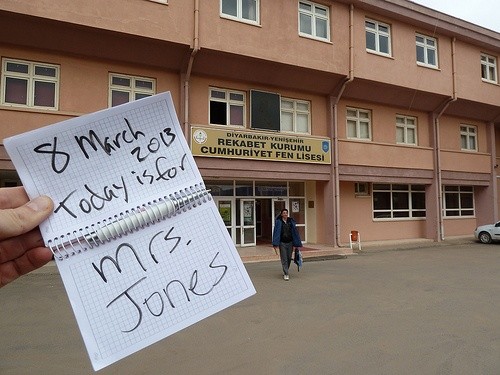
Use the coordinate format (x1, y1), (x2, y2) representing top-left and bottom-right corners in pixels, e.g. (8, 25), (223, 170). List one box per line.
(474, 220), (500, 243)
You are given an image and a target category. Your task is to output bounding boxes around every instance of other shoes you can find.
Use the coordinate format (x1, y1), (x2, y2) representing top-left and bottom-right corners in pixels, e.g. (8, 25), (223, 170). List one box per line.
(282, 271), (290, 280)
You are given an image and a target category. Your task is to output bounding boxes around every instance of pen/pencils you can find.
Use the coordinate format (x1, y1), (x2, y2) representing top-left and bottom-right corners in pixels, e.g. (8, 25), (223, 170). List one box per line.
(84, 188), (213, 242)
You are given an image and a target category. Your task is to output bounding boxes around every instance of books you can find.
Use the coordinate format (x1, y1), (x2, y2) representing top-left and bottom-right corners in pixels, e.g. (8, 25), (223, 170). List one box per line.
(3, 90), (257, 371)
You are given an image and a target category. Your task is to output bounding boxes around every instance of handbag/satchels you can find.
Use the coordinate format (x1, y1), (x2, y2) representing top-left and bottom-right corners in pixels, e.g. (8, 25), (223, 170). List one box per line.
(294, 249), (303, 272)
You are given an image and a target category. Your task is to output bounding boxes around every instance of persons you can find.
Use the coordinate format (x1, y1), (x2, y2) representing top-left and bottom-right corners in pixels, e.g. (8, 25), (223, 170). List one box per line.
(272, 209), (302, 282)
(0, 186), (55, 291)
